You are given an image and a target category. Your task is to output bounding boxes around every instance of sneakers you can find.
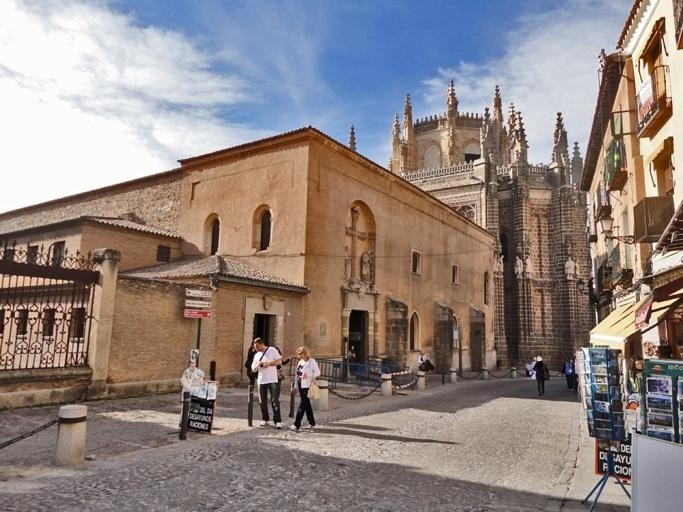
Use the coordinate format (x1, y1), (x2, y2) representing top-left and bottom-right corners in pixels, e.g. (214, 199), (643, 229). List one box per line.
(259, 419), (315, 431)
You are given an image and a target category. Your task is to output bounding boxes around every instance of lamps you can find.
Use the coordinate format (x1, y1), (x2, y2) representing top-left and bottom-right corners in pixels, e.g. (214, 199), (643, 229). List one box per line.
(598, 202), (635, 244)
(577, 278), (589, 295)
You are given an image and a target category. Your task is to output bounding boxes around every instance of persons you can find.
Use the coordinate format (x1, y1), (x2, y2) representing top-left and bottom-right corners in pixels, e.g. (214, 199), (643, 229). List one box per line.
(563, 256), (575, 280)
(250, 338), (283, 429)
(267, 346), (290, 398)
(561, 354), (576, 393)
(574, 259), (581, 279)
(494, 253), (533, 281)
(417, 350), (425, 367)
(245, 342), (257, 385)
(532, 356), (547, 396)
(287, 345), (321, 432)
(347, 345), (356, 363)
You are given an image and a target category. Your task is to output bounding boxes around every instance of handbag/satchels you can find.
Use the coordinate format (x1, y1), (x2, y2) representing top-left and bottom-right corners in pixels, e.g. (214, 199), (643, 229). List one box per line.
(307, 385), (320, 399)
